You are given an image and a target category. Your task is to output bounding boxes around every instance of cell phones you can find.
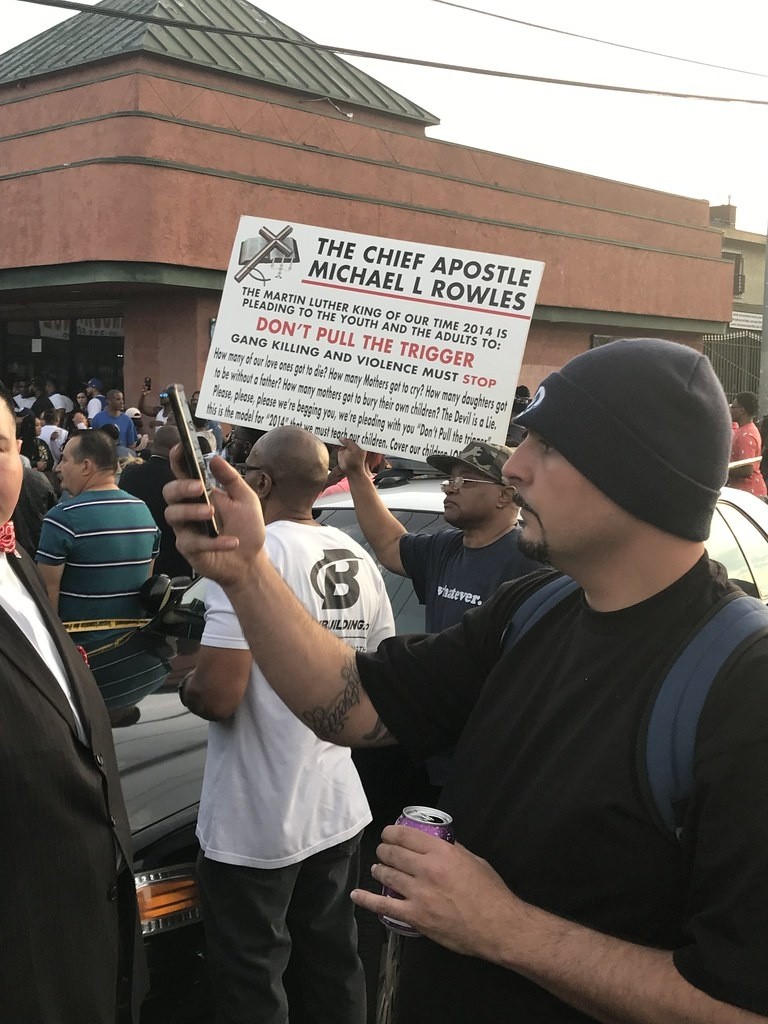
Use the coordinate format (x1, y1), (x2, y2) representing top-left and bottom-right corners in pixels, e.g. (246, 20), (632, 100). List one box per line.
(166, 383), (223, 538)
(144, 377), (152, 391)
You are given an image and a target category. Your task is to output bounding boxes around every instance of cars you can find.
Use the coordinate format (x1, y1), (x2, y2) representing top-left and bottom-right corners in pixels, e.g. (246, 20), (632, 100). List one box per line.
(102, 459), (767, 989)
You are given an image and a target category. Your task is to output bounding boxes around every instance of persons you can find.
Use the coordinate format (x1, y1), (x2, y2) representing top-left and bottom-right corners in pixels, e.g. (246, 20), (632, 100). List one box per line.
(0, 381), (205, 1024)
(318, 443), (383, 499)
(162, 337), (768, 1023)
(181, 425), (395, 1024)
(729, 391), (768, 499)
(35, 429), (160, 649)
(338, 438), (547, 635)
(11, 376), (266, 557)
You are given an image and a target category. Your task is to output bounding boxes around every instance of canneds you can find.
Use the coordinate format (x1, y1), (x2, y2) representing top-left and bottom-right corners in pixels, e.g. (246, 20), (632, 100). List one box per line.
(378, 805), (453, 938)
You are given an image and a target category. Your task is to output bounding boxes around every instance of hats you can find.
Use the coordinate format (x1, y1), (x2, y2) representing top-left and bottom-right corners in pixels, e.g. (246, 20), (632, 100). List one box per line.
(512, 338), (732, 542)
(125, 407), (141, 418)
(516, 385), (530, 397)
(80, 378), (103, 391)
(426, 441), (515, 486)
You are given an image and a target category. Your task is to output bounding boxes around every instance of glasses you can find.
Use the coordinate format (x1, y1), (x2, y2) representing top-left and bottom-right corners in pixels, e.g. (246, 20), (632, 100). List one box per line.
(729, 404), (742, 409)
(440, 477), (506, 492)
(515, 396), (528, 401)
(159, 392), (169, 398)
(234, 463), (277, 486)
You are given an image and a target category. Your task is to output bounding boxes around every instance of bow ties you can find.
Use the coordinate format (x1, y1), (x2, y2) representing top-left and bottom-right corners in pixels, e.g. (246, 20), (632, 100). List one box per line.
(0, 521), (15, 553)
